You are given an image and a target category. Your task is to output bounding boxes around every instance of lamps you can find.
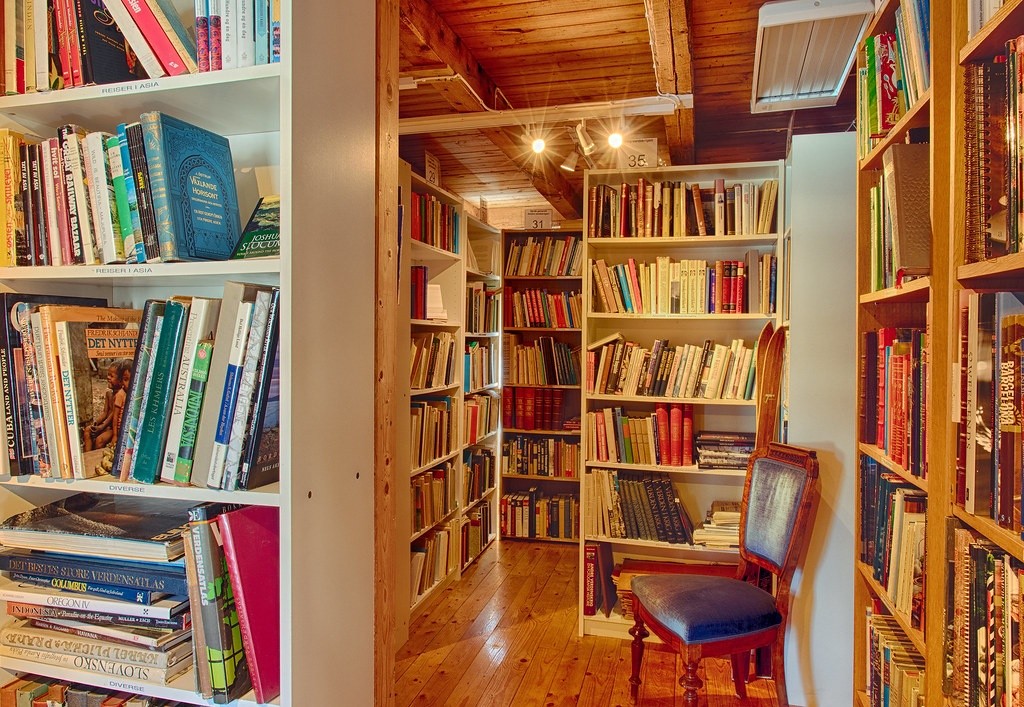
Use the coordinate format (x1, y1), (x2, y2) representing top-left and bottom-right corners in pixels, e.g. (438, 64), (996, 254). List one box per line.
(750, 0), (874, 115)
(559, 151), (579, 171)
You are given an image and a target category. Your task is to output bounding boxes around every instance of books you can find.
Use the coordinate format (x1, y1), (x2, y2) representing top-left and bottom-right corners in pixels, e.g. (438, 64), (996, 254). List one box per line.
(588, 176), (778, 238)
(588, 249), (777, 314)
(0, 110), (280, 267)
(501, 234), (583, 540)
(586, 402), (756, 471)
(584, 468), (741, 554)
(586, 332), (759, 401)
(858, 0), (1024, 707)
(584, 541), (738, 623)
(0, 492), (280, 707)
(411, 191), (500, 610)
(0, 0), (280, 97)
(0, 280), (280, 492)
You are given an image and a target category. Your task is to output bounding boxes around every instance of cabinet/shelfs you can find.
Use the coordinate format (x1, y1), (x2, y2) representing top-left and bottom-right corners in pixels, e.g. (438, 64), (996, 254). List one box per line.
(0, 0), (1024, 707)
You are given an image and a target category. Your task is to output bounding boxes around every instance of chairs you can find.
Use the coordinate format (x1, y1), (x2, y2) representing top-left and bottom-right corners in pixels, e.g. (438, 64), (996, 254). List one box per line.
(630, 441), (821, 707)
